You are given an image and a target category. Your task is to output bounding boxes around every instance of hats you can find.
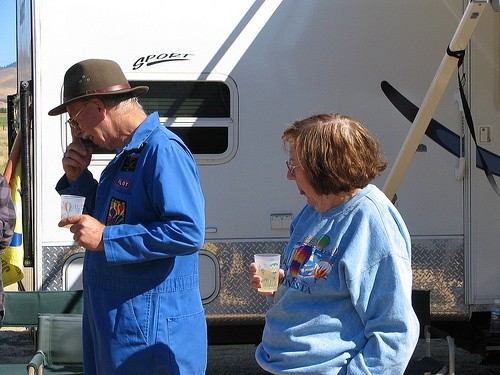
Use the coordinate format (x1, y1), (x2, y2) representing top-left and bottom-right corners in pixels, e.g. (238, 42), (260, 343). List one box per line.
(48, 59), (149, 116)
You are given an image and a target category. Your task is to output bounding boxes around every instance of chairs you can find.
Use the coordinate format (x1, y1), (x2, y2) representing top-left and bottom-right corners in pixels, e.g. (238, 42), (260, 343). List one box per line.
(27, 313), (84, 375)
(403, 290), (455, 375)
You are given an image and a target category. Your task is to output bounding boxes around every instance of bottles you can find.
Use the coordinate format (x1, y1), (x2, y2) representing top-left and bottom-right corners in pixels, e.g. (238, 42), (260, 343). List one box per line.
(489, 300), (500, 332)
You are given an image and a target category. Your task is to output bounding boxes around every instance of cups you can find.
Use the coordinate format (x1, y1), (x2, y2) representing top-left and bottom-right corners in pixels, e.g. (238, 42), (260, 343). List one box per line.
(61, 195), (85, 228)
(254, 255), (280, 293)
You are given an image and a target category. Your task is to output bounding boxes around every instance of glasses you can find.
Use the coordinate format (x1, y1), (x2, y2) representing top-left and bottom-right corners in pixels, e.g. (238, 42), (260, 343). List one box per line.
(286, 161), (301, 173)
(68, 103), (87, 128)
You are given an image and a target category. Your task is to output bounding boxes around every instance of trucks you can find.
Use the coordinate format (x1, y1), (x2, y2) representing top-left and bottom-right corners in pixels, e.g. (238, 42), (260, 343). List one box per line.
(0, 1), (500, 375)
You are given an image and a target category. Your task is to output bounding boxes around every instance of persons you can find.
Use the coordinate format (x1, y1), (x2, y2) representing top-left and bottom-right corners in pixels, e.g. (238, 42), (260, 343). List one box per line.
(0, 170), (16, 328)
(54, 58), (208, 375)
(250, 112), (421, 375)
